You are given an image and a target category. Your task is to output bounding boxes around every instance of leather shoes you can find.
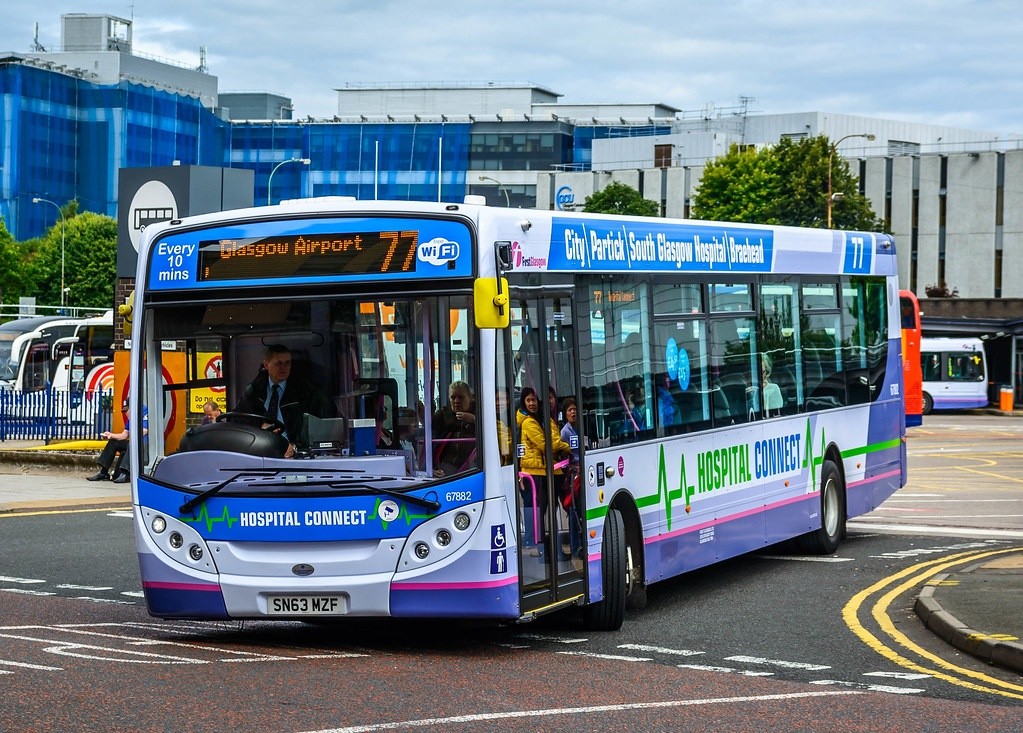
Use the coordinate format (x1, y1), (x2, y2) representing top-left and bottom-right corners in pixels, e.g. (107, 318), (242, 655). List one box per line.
(86, 471), (110, 481)
(114, 472), (130, 483)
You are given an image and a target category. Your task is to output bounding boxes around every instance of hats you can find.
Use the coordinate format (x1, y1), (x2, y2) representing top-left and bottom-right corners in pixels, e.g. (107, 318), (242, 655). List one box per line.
(121, 397), (129, 412)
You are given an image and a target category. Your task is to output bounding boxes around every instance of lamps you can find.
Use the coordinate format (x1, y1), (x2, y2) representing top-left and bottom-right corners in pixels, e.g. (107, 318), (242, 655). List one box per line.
(496, 114), (504, 122)
(360, 115), (368, 122)
(938, 152), (949, 158)
(637, 168), (644, 172)
(469, 114), (476, 122)
(884, 156), (894, 161)
(552, 114), (558, 121)
(620, 117), (625, 125)
(660, 167), (667, 170)
(333, 115), (341, 122)
(591, 116), (599, 125)
(683, 166), (691, 170)
(387, 115), (395, 122)
(441, 114), (449, 122)
(967, 152), (980, 158)
(26, 57), (99, 79)
(995, 150), (1006, 155)
(648, 117), (654, 125)
(414, 114), (422, 122)
(592, 171), (599, 174)
(549, 173), (555, 177)
(858, 156), (867, 161)
(524, 114), (531, 122)
(602, 170), (612, 175)
(910, 155), (921, 160)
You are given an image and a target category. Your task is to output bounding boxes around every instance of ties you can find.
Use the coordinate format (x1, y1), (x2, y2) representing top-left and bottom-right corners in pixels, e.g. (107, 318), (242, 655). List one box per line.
(267, 385), (280, 419)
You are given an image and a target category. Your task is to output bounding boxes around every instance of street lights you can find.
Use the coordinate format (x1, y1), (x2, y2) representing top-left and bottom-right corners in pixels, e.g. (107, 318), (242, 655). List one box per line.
(33, 197), (64, 315)
(827, 133), (875, 229)
(267, 157), (312, 206)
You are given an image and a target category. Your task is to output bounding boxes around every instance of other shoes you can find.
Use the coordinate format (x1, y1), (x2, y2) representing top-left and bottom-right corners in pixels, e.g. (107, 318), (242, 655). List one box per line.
(562, 544), (570, 554)
(572, 559), (583, 573)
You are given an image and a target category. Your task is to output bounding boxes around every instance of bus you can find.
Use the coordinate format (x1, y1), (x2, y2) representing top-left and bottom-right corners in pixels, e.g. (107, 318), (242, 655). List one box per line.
(0, 196), (924, 632)
(920, 336), (988, 415)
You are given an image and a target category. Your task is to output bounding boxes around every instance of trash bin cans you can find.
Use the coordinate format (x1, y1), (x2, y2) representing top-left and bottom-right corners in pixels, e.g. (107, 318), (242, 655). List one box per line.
(1000, 384), (1014, 412)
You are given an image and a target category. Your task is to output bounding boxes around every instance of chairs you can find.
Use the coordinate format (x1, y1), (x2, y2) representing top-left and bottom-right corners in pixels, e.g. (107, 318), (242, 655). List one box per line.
(670, 383), (702, 424)
(808, 331), (837, 377)
(769, 366), (796, 407)
(712, 384), (731, 418)
(720, 373), (749, 416)
(308, 115), (315, 123)
(802, 339), (823, 398)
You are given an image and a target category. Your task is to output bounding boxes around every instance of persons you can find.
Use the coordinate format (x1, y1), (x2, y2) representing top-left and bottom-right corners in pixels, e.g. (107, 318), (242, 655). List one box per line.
(229, 345), (338, 458)
(201, 400), (227, 426)
(614, 381), (681, 434)
(496, 387), (573, 542)
(433, 380), (477, 476)
(748, 352), (783, 411)
(395, 406), (445, 478)
(87, 397), (149, 484)
(561, 397), (580, 462)
(379, 405), (403, 450)
